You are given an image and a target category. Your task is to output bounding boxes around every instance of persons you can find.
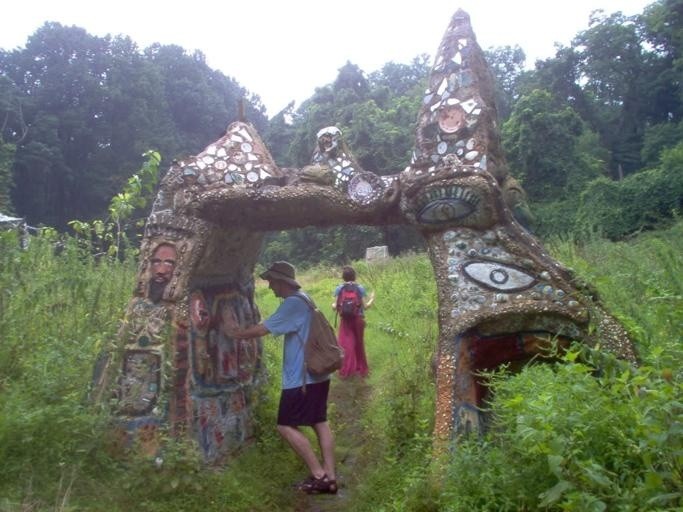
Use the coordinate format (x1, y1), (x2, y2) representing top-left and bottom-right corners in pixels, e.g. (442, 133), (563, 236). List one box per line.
(222, 262), (337, 494)
(332, 267), (375, 380)
(147, 243), (178, 305)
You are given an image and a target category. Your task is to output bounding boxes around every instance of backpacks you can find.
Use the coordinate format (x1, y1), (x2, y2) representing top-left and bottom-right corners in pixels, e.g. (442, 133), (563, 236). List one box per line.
(334, 283), (363, 329)
(284, 292), (345, 395)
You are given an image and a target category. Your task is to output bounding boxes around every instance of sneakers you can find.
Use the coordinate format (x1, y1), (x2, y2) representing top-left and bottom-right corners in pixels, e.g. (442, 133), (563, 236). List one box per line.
(293, 472), (339, 495)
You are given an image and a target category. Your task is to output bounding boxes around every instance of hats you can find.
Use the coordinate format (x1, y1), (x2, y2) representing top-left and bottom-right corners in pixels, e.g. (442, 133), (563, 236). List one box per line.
(259, 260), (303, 290)
